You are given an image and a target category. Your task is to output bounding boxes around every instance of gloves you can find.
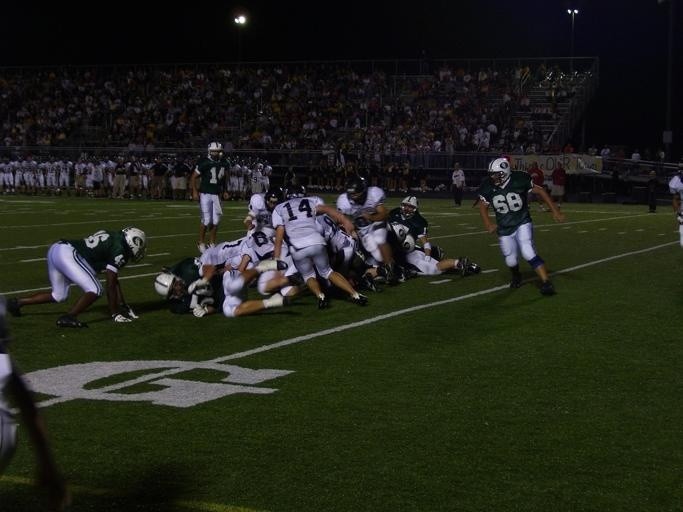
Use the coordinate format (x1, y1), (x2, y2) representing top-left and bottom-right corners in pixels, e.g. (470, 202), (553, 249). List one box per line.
(112, 312), (131, 322)
(121, 302), (138, 318)
(194, 304), (207, 317)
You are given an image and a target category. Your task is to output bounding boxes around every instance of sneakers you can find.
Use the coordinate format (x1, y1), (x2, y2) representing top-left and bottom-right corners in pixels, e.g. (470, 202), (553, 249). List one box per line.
(258, 256), (480, 309)
(57, 315), (87, 328)
(6, 298), (21, 317)
(541, 280), (554, 296)
(198, 242), (206, 253)
(511, 273), (522, 289)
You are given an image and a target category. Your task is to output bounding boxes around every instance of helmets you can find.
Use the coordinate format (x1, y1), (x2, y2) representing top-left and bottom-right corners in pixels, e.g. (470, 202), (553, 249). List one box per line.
(487, 158), (512, 187)
(155, 273), (186, 300)
(265, 177), (418, 251)
(207, 142), (222, 164)
(121, 226), (147, 263)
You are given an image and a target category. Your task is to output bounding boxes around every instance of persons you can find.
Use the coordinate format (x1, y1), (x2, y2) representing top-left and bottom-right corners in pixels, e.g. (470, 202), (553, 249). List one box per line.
(478, 157), (566, 296)
(0, 291), (73, 512)
(1, 60), (683, 213)
(668, 159), (683, 249)
(5, 227), (147, 327)
(153, 176), (481, 319)
